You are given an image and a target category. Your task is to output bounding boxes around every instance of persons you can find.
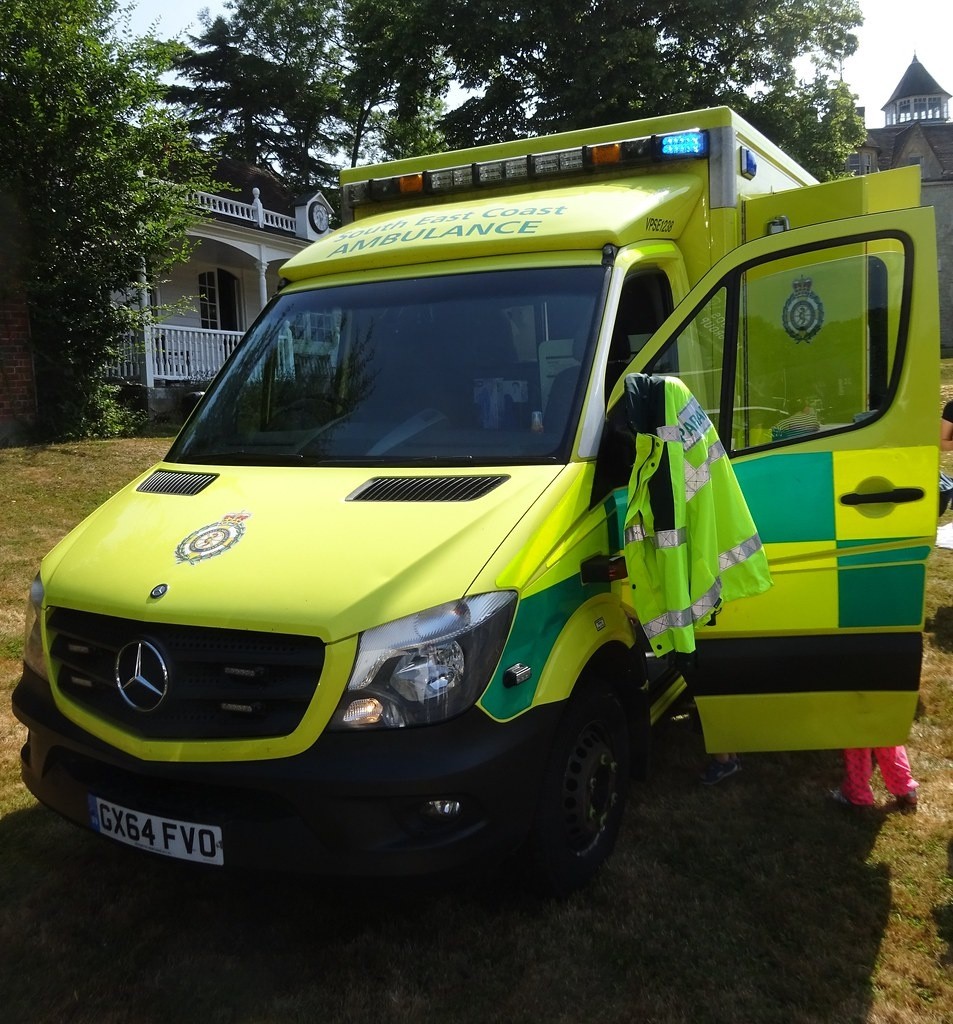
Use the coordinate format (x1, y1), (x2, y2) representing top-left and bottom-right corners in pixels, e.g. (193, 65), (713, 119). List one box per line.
(826, 745), (918, 812)
(770, 395), (821, 441)
(698, 752), (742, 785)
(939, 398), (953, 453)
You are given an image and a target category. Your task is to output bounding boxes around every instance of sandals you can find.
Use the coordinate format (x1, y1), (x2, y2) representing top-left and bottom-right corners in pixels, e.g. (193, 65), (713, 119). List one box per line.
(697, 759), (738, 786)
(730, 758), (742, 772)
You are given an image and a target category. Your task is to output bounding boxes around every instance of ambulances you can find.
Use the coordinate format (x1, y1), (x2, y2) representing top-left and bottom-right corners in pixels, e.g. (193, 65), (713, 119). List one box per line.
(10, 106), (939, 912)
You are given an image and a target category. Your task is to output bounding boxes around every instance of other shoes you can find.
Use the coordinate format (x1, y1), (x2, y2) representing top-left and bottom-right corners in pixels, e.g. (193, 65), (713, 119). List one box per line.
(848, 802), (873, 819)
(897, 795), (917, 808)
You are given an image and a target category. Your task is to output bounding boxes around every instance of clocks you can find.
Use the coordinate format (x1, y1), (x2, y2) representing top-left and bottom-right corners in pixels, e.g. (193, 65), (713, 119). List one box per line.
(309, 200), (329, 234)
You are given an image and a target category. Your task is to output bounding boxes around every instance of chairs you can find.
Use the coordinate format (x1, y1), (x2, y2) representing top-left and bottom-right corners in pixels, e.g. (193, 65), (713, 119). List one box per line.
(155, 334), (190, 375)
